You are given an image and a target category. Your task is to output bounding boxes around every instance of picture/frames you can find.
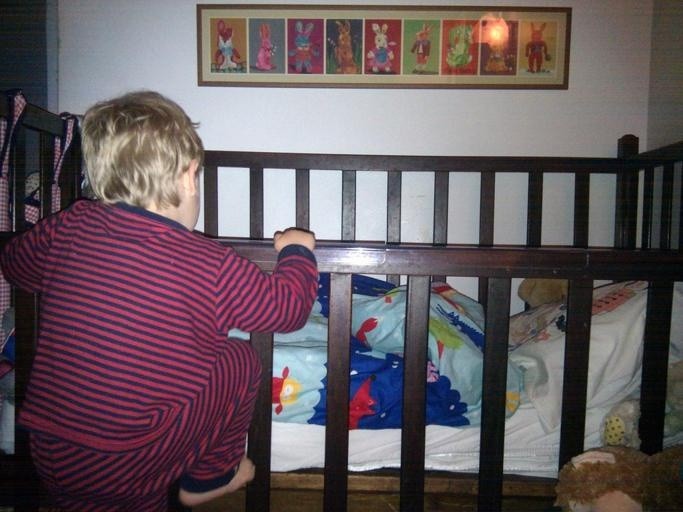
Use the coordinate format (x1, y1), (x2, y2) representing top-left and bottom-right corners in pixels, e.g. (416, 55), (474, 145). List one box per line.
(194, 4), (571, 90)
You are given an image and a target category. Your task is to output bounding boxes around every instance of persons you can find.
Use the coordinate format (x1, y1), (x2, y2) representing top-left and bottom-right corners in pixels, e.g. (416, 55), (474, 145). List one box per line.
(0, 89), (319, 512)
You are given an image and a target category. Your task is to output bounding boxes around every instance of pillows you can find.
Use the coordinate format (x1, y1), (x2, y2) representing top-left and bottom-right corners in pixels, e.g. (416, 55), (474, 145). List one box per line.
(508, 280), (683, 436)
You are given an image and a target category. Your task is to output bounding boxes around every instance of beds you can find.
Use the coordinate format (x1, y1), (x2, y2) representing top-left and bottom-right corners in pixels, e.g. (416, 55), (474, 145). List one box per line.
(1, 89), (682, 510)
